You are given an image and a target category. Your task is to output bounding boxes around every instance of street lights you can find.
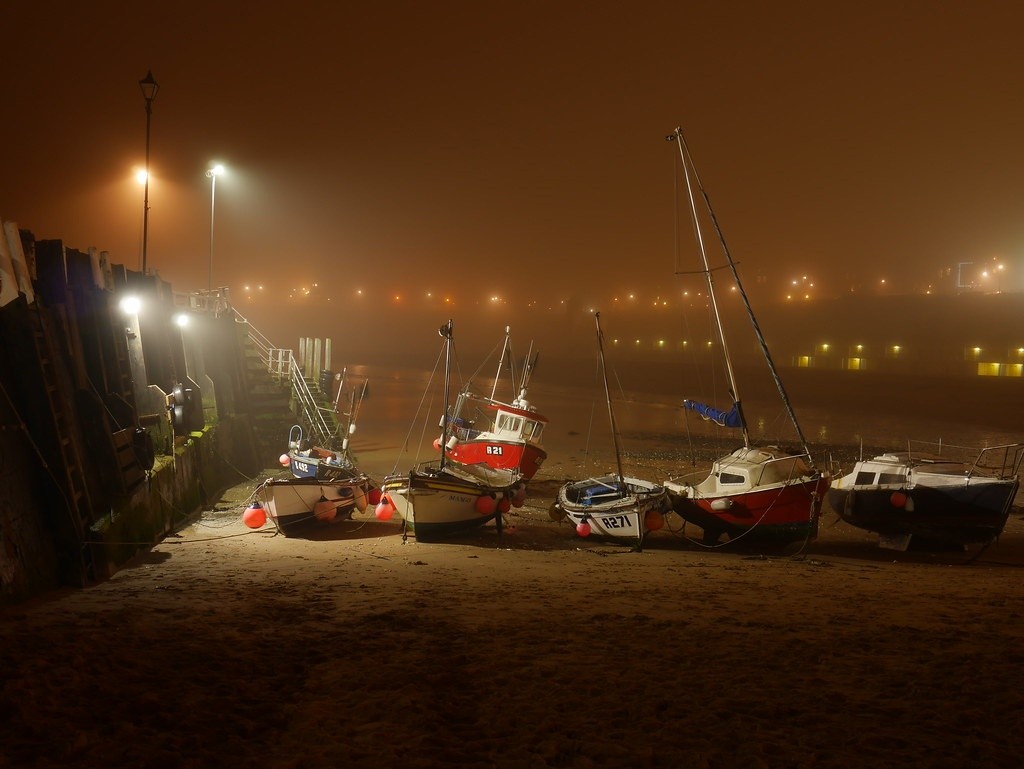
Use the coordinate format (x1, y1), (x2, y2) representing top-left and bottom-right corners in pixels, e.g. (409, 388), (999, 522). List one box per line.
(208, 162), (227, 290)
(136, 69), (160, 275)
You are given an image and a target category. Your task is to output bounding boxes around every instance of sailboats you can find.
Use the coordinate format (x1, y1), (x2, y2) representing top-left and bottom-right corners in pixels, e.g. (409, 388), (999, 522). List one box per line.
(557, 311), (665, 546)
(381, 319), (528, 543)
(667, 123), (832, 548)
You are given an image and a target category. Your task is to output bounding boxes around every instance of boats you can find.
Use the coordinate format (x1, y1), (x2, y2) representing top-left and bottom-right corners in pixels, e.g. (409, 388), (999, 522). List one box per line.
(254, 378), (369, 535)
(433, 325), (548, 486)
(827, 444), (1024, 549)
